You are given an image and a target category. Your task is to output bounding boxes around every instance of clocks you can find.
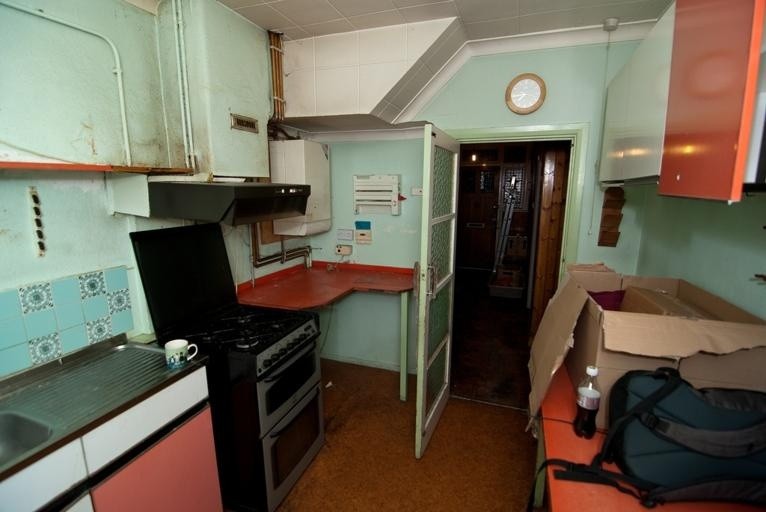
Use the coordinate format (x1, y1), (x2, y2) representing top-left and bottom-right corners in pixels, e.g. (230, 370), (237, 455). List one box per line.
(505, 72), (547, 115)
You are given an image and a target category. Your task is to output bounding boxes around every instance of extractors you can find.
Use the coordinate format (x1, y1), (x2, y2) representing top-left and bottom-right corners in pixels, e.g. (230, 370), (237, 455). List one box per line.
(145, 181), (312, 224)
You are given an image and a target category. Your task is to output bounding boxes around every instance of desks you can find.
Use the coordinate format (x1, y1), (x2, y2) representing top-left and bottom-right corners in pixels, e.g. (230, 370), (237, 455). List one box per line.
(236, 260), (416, 403)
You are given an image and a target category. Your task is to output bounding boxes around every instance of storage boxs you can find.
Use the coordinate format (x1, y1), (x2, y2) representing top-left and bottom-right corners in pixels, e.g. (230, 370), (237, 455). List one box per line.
(566, 265), (616, 272)
(619, 287), (722, 320)
(526, 268), (766, 432)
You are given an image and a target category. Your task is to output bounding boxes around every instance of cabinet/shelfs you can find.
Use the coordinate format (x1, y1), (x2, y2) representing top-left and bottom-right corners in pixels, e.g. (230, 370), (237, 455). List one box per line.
(269, 139), (332, 236)
(0, 423), (95, 512)
(89, 357), (225, 512)
(596, 1), (766, 206)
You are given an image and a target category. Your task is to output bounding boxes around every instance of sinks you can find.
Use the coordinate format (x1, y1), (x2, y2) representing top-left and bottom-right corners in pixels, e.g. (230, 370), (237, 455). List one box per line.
(0, 404), (63, 479)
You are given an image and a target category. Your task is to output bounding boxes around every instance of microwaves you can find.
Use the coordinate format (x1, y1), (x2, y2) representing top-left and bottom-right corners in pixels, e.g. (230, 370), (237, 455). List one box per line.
(256, 335), (321, 439)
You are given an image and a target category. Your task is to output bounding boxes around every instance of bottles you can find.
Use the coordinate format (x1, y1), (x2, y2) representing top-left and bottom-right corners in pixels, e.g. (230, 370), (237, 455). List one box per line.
(572, 366), (601, 439)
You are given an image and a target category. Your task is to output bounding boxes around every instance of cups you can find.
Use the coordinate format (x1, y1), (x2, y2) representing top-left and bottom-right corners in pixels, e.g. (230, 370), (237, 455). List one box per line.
(165, 338), (197, 368)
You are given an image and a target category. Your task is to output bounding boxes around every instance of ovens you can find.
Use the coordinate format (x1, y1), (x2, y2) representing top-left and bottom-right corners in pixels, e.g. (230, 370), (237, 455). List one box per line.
(260, 382), (324, 512)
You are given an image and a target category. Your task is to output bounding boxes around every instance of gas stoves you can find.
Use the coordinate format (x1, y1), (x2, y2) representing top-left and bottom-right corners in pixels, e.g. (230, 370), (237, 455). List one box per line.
(161, 302), (321, 360)
